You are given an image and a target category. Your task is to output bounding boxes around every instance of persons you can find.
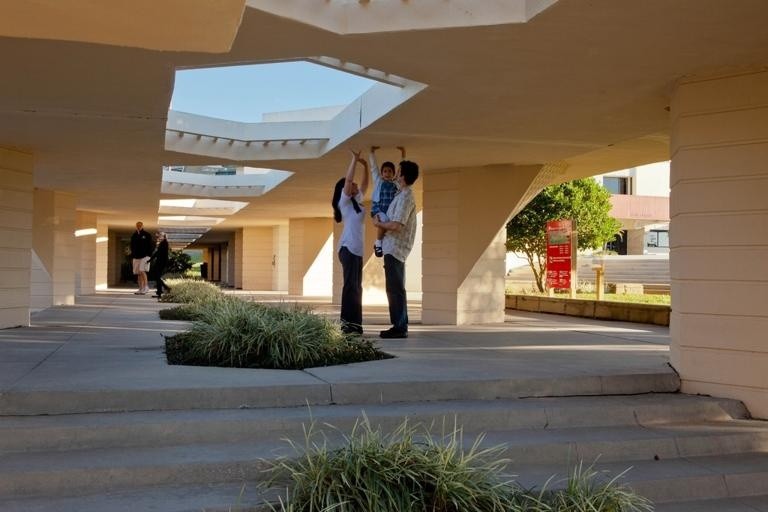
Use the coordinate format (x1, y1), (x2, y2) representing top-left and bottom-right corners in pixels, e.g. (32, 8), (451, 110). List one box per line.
(372, 146), (406, 258)
(137, 260), (150, 292)
(148, 232), (171, 299)
(331, 145), (369, 341)
(131, 222), (152, 294)
(371, 161), (418, 338)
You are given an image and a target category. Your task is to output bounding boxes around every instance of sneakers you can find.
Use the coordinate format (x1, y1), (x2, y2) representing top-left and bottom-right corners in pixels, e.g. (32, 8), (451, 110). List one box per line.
(135, 288), (148, 295)
(379, 325), (408, 339)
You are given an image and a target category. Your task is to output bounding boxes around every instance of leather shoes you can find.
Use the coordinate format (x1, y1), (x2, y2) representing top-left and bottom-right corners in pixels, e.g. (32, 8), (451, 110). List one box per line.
(374, 244), (383, 257)
